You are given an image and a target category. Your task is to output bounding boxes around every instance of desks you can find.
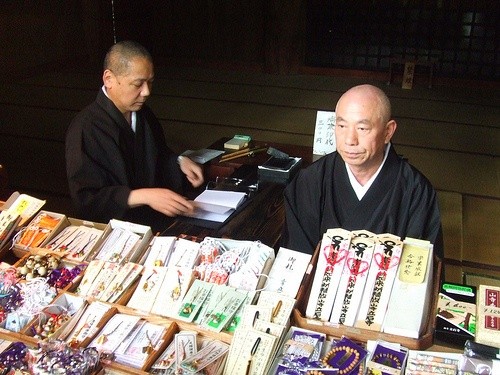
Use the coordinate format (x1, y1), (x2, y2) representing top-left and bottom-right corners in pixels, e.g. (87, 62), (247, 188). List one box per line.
(164, 134), (314, 253)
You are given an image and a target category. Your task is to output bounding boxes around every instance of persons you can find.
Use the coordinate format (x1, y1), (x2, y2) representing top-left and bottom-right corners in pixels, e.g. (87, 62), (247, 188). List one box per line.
(285, 84), (447, 285)
(67, 41), (205, 230)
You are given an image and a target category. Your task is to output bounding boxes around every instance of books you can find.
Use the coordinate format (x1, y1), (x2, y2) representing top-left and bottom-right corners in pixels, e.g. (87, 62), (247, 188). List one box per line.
(183, 188), (251, 227)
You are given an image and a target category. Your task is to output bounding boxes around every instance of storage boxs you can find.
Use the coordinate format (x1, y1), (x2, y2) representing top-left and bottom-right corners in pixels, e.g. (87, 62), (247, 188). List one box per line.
(0, 194), (477, 375)
